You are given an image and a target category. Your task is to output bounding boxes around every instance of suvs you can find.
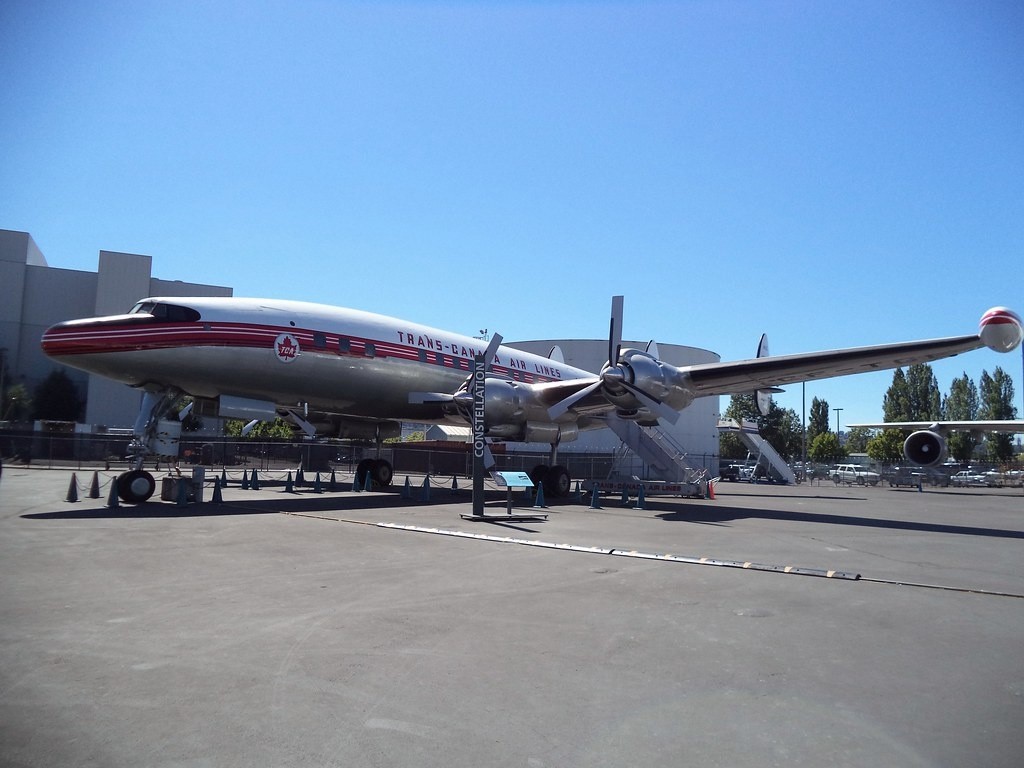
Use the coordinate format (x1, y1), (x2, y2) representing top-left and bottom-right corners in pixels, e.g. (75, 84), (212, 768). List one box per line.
(885, 465), (952, 488)
(829, 464), (880, 486)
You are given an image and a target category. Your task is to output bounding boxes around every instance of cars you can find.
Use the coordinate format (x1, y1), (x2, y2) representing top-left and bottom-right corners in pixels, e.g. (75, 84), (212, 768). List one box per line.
(982, 468), (1024, 480)
(720, 460), (828, 482)
(337, 454), (360, 464)
(949, 470), (986, 484)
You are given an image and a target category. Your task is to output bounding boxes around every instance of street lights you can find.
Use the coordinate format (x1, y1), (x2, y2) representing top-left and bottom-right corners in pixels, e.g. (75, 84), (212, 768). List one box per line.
(832, 408), (844, 440)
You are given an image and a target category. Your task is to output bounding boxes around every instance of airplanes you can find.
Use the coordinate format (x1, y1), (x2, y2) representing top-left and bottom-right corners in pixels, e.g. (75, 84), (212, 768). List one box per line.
(37, 294), (1024, 505)
(849, 420), (1023, 465)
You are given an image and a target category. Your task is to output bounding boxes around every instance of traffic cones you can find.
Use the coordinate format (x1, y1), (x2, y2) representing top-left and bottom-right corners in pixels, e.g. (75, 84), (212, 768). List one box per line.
(708, 480), (716, 499)
